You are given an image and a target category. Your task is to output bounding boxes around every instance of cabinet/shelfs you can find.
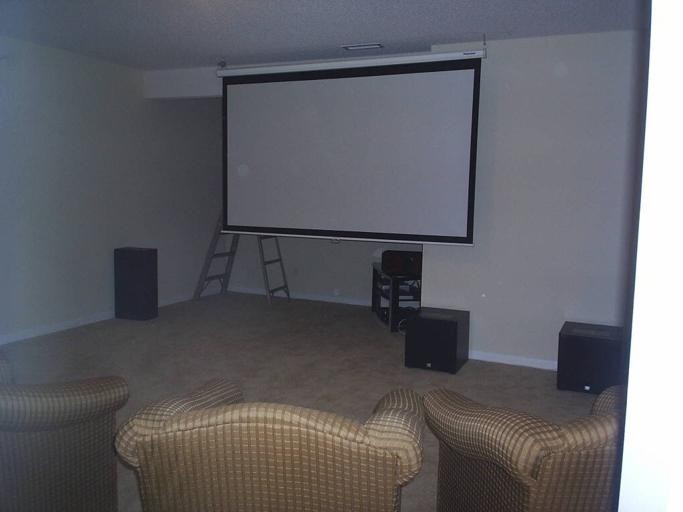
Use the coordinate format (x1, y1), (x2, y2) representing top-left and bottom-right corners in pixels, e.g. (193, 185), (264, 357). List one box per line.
(372, 262), (421, 332)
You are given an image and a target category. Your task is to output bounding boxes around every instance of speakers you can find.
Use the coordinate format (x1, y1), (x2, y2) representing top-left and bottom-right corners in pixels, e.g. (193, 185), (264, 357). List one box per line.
(405, 306), (470, 375)
(557, 320), (625, 395)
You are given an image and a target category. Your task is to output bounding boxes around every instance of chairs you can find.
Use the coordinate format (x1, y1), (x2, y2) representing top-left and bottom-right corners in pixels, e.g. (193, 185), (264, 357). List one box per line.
(423, 383), (627, 512)
(0, 353), (130, 512)
(114, 374), (425, 512)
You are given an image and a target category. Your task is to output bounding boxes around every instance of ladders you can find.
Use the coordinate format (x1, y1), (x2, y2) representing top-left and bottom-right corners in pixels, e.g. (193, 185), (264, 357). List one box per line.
(193, 208), (291, 306)
